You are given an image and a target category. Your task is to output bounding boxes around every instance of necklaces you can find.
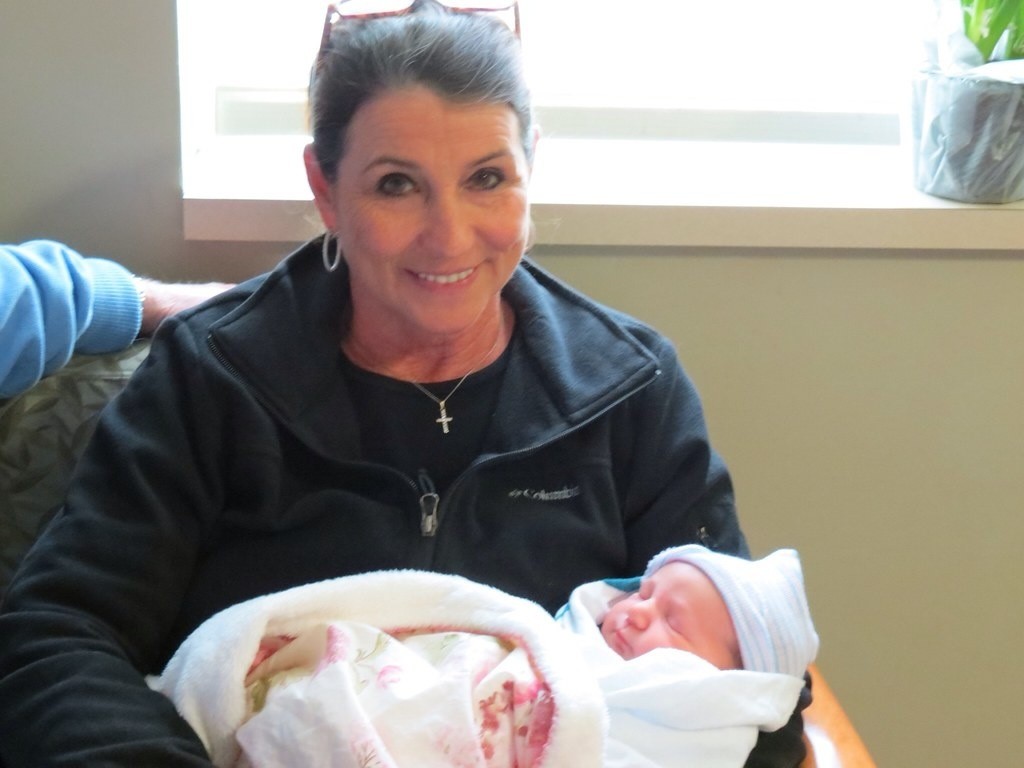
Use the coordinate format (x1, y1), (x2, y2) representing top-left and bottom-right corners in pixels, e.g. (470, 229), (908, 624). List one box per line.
(399, 311), (506, 433)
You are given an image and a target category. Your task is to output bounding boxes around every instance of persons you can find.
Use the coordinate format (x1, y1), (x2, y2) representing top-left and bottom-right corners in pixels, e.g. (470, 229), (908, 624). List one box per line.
(0, 4), (816, 768)
(0, 239), (240, 392)
(139, 550), (812, 768)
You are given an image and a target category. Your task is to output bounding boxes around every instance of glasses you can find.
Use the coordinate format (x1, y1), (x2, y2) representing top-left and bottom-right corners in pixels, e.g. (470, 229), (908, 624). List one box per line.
(318, 0), (523, 53)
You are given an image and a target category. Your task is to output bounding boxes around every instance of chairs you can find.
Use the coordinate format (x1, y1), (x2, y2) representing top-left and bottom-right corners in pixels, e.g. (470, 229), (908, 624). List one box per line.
(0, 337), (877, 768)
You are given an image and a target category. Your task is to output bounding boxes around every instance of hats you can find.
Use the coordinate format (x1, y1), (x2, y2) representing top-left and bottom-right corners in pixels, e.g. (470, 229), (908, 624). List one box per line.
(639, 543), (819, 676)
